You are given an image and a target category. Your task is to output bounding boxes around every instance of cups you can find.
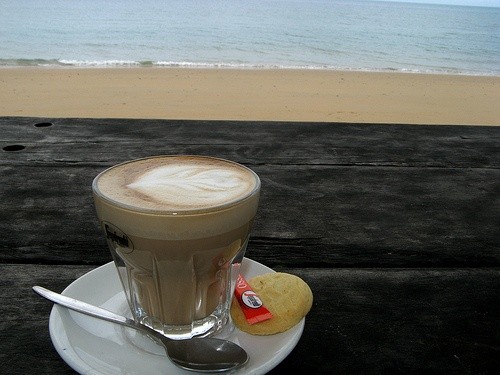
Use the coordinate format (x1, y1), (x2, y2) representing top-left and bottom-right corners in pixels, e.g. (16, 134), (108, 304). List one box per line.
(92, 154), (262, 340)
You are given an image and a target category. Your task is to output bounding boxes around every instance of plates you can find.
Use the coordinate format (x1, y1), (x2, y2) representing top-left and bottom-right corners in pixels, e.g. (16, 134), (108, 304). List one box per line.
(48, 256), (306, 375)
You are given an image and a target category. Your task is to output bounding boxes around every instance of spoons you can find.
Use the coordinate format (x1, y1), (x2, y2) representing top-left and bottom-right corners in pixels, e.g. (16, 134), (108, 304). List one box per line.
(32, 285), (250, 372)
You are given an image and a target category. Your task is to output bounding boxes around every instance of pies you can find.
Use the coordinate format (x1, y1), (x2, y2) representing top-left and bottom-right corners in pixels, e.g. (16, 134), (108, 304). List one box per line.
(230, 272), (313, 336)
